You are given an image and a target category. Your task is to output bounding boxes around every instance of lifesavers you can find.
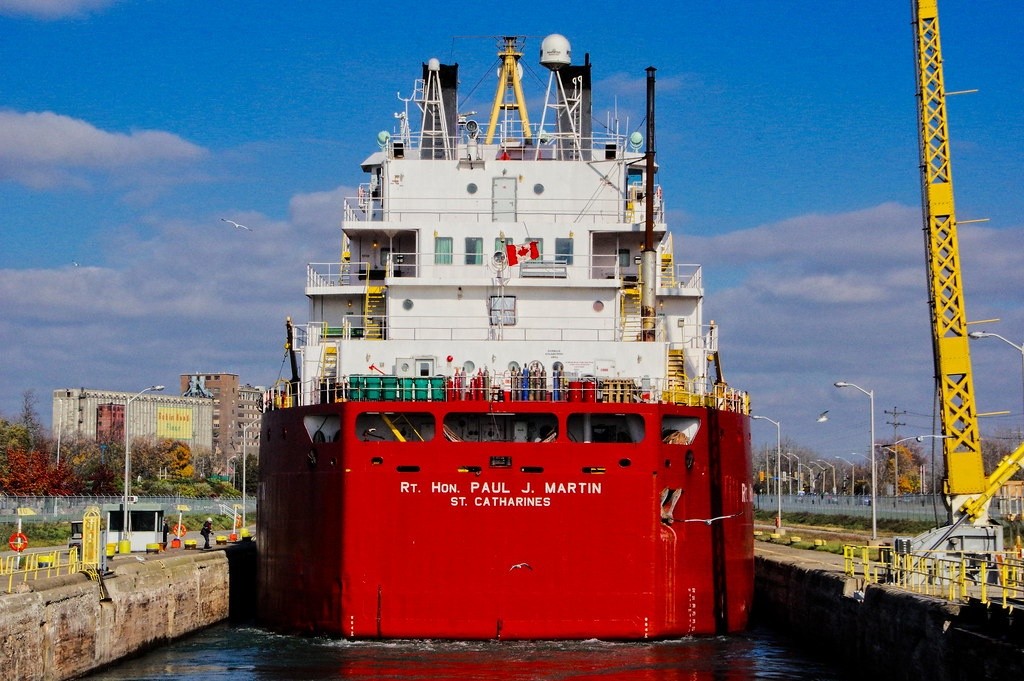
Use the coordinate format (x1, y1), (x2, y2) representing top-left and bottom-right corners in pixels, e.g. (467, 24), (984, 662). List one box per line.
(9, 533), (28, 552)
(235, 516), (241, 527)
(173, 524), (187, 537)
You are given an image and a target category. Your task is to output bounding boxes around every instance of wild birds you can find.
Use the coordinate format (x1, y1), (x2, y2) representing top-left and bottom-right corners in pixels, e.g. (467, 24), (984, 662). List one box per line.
(881, 435), (959, 447)
(72, 260), (79, 267)
(221, 218), (249, 232)
(670, 511), (743, 525)
(510, 563), (532, 571)
(816, 411), (829, 423)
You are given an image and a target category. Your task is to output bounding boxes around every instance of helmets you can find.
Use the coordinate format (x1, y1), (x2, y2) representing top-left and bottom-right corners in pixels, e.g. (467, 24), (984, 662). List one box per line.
(206, 517), (213, 521)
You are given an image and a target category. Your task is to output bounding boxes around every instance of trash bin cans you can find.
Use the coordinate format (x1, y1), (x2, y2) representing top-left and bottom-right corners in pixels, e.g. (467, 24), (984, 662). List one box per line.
(414, 377), (428, 401)
(429, 376), (445, 400)
(349, 374), (364, 401)
(382, 374), (399, 401)
(366, 374), (381, 401)
(397, 377), (413, 401)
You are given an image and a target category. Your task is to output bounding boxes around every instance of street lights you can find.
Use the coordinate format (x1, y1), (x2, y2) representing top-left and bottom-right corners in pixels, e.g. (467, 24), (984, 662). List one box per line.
(835, 456), (854, 495)
(834, 381), (877, 540)
(752, 416), (781, 528)
(123, 385), (165, 540)
(787, 453), (799, 491)
(777, 453), (791, 495)
(868, 443), (898, 496)
(798, 463), (812, 487)
(817, 459), (835, 493)
(810, 461), (825, 493)
(851, 453), (878, 496)
(968, 332), (1024, 375)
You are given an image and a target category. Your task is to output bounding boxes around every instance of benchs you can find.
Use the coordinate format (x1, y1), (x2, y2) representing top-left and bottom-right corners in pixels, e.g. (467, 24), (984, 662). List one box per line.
(519, 258), (568, 278)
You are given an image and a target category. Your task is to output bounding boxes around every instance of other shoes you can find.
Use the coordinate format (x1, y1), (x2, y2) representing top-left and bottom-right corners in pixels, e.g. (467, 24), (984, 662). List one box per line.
(203, 546), (213, 551)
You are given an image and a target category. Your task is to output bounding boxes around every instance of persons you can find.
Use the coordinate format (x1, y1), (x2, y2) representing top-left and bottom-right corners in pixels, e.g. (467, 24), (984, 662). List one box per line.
(163, 519), (169, 542)
(810, 492), (831, 505)
(537, 128), (547, 145)
(200, 517), (214, 549)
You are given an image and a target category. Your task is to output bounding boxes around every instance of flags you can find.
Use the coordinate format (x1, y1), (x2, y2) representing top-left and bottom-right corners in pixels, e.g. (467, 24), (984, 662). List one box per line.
(506, 242), (539, 266)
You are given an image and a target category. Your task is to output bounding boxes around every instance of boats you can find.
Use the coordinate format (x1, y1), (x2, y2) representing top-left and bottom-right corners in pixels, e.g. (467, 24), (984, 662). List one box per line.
(252, 33), (757, 642)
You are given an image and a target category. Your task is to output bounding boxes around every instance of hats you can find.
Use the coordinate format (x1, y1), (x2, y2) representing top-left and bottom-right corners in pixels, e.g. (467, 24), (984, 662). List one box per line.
(163, 519), (169, 524)
